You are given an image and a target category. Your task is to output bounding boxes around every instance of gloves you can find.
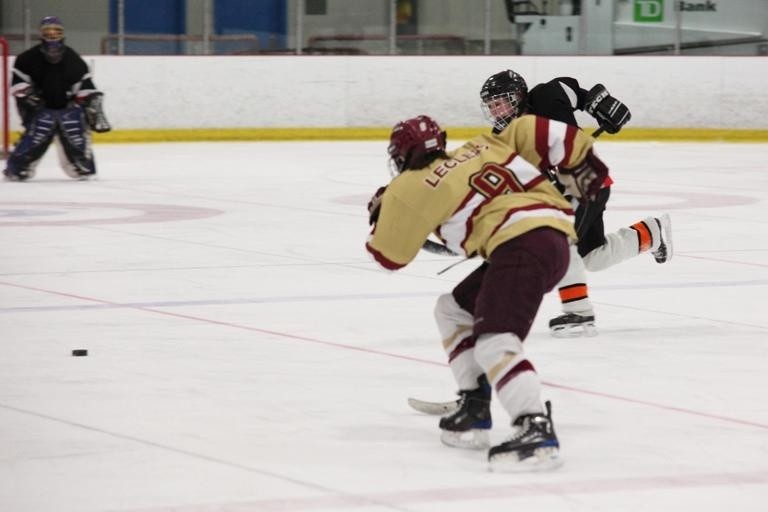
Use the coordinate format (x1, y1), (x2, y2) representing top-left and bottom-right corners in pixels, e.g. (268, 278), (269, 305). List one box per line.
(366, 184), (389, 225)
(559, 148), (609, 204)
(583, 83), (631, 134)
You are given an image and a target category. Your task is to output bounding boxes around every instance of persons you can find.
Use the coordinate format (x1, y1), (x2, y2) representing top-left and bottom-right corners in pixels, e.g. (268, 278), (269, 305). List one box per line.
(3, 17), (111, 181)
(479, 69), (669, 329)
(364, 114), (609, 461)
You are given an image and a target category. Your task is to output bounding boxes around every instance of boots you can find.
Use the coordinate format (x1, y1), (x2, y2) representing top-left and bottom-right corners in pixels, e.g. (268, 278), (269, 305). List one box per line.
(488, 400), (560, 459)
(440, 373), (493, 431)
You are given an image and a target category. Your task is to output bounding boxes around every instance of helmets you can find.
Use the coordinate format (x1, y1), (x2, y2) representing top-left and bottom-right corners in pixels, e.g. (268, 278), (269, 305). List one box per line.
(387, 115), (446, 175)
(479, 69), (527, 130)
(39, 16), (65, 55)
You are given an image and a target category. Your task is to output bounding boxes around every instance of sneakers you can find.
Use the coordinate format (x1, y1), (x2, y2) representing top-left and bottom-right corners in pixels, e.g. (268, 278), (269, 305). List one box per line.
(548, 312), (595, 329)
(651, 218), (667, 263)
